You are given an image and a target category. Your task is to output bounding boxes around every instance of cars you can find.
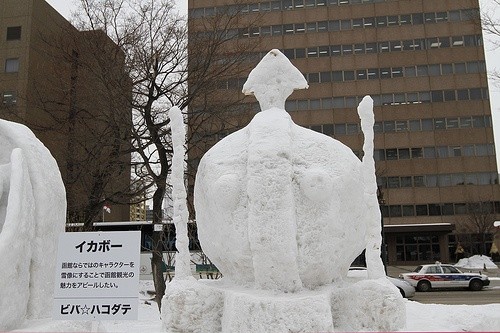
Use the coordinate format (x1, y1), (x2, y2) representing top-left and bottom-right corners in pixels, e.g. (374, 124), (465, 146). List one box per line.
(398, 264), (490, 292)
(386, 275), (417, 298)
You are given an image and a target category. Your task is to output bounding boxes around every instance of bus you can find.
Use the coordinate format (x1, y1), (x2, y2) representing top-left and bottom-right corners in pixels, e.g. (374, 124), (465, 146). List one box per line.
(67, 218), (220, 273)
